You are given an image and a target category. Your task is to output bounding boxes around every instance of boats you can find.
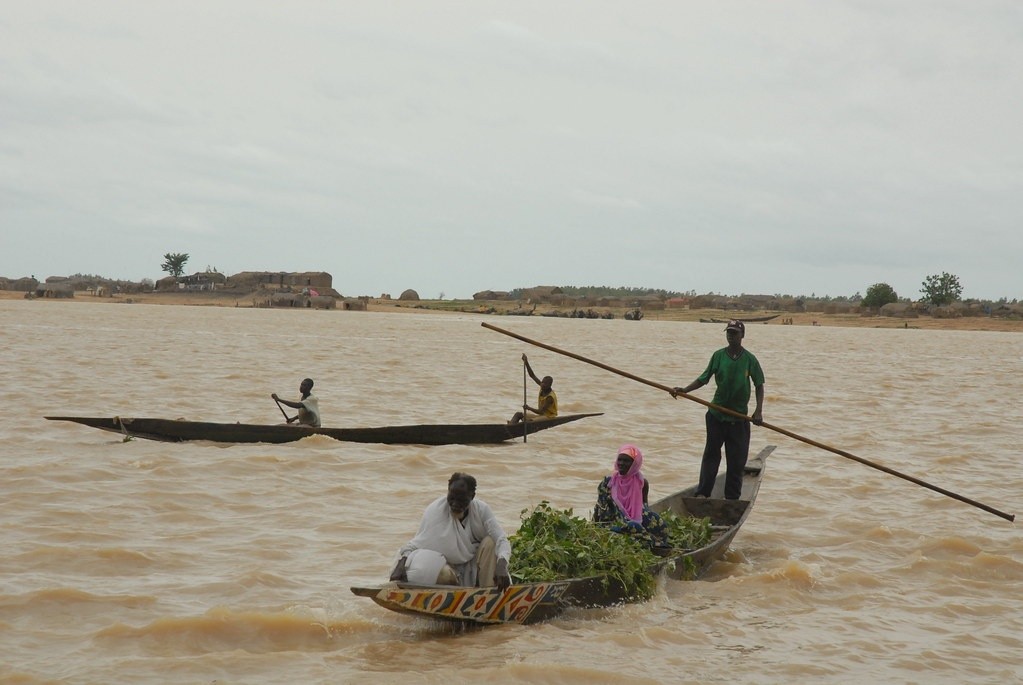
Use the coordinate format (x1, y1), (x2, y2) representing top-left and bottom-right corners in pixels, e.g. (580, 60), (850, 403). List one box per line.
(350, 443), (793, 629)
(42, 410), (608, 446)
(729, 314), (781, 322)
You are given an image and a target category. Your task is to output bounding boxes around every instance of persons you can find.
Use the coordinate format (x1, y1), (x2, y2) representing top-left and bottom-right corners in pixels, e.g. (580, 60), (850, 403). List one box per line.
(594, 445), (667, 546)
(670, 320), (765, 500)
(508, 353), (558, 424)
(390, 473), (511, 593)
(271, 378), (321, 427)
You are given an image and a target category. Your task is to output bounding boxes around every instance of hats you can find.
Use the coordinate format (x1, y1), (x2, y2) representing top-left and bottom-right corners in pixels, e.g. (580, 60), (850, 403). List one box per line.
(724, 320), (745, 332)
